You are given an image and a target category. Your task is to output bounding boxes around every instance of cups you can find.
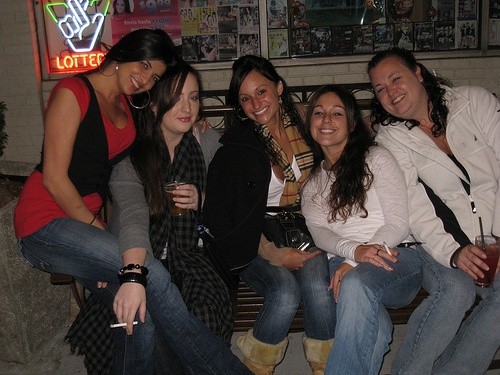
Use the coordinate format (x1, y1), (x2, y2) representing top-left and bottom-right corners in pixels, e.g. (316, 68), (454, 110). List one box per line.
(164, 182), (190, 217)
(473, 235), (500, 288)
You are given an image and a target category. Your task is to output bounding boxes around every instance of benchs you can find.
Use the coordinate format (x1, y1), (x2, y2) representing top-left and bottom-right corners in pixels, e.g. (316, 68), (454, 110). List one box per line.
(49, 81), (482, 333)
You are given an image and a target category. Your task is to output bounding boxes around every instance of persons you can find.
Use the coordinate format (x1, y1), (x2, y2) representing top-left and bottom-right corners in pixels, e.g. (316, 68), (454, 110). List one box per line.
(227, 53), (338, 374)
(366, 47), (500, 375)
(13, 28), (253, 375)
(112, 0), (134, 16)
(62, 61), (235, 375)
(177, 0), (500, 61)
(299, 85), (424, 375)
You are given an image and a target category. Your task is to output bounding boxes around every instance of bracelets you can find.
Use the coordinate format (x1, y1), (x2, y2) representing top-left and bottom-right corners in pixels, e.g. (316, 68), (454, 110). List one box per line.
(119, 272), (147, 289)
(119, 264), (149, 276)
(89, 216), (96, 225)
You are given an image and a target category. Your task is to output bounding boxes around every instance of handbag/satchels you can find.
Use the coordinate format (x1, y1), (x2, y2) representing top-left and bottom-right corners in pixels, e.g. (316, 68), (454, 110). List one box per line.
(261, 211), (315, 252)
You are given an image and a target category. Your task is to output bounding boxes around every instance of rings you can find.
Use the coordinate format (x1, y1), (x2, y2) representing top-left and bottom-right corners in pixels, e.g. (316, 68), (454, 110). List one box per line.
(377, 249), (380, 255)
(338, 280), (341, 283)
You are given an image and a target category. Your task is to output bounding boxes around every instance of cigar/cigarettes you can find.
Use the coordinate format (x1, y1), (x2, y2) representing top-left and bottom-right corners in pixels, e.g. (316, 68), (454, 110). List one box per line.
(110, 321), (138, 328)
(383, 241), (392, 256)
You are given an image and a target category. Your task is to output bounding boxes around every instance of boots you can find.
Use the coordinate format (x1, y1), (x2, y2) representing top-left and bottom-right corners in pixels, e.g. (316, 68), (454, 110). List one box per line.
(234, 326), (289, 375)
(302, 333), (335, 375)
